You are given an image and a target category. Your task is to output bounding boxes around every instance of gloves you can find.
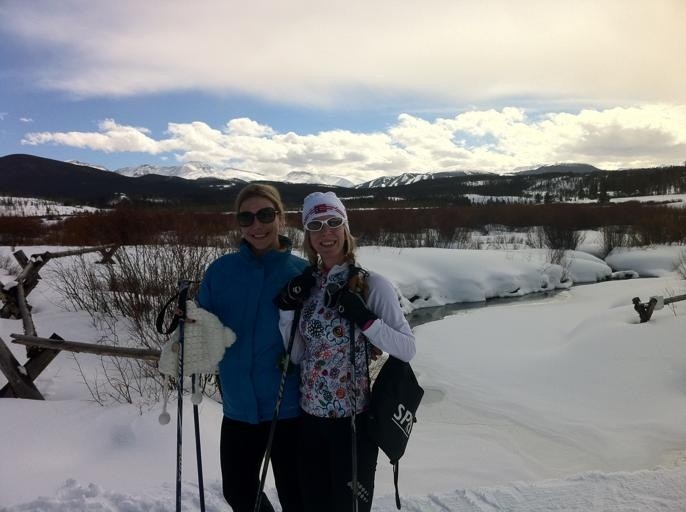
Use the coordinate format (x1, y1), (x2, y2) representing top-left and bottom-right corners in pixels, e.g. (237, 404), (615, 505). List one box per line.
(274, 274), (315, 311)
(332, 286), (376, 327)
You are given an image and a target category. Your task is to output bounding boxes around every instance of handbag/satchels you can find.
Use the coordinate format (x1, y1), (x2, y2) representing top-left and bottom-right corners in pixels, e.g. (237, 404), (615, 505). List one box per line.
(355, 353), (424, 464)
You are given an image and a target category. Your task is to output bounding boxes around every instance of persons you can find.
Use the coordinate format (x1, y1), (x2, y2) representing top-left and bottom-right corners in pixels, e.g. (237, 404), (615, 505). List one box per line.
(270, 189), (417, 512)
(170, 184), (310, 512)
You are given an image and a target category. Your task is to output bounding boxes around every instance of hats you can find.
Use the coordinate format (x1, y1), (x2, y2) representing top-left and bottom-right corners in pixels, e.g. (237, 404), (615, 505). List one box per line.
(301, 191), (349, 228)
(158, 300), (236, 377)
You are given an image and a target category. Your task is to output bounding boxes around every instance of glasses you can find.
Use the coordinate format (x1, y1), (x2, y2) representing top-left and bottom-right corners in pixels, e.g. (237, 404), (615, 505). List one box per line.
(304, 217), (345, 231)
(237, 207), (279, 227)
